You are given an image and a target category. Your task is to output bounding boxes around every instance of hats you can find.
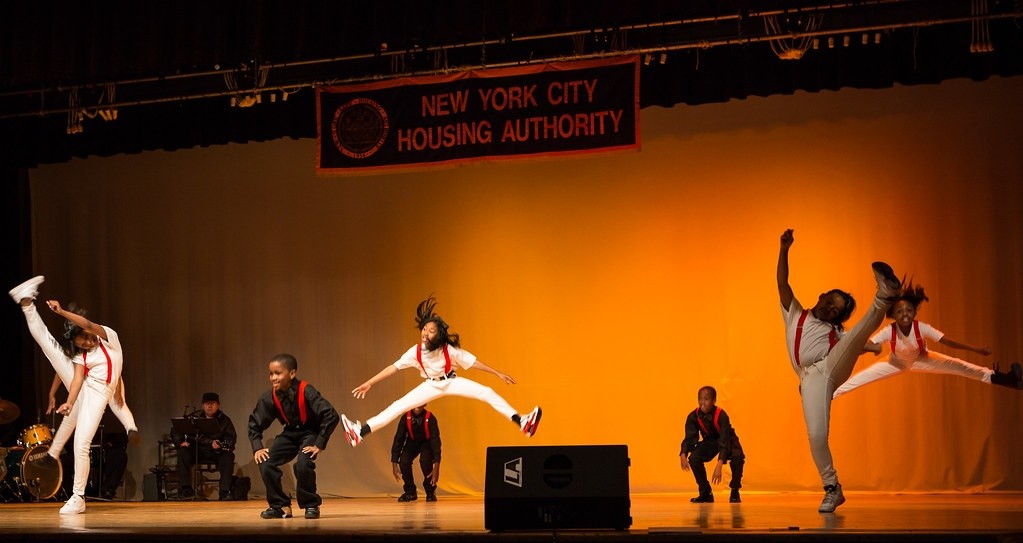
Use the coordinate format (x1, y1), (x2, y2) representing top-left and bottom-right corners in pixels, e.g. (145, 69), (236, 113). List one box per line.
(203, 393), (219, 403)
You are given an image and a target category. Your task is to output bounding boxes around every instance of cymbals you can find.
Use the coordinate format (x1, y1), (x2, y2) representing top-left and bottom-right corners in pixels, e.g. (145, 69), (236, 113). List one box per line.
(0, 398), (22, 425)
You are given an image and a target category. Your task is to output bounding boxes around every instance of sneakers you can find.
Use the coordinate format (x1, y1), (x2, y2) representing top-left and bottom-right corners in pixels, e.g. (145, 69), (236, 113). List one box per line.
(341, 414), (364, 447)
(59, 494), (85, 514)
(872, 262), (902, 299)
(819, 483), (845, 513)
(520, 406), (542, 437)
(9, 276), (44, 303)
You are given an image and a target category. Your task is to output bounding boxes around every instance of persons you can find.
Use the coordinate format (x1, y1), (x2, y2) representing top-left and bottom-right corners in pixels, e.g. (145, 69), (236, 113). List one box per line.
(177, 392), (237, 501)
(247, 353), (340, 519)
(391, 403), (442, 502)
(679, 386), (746, 503)
(776, 228), (902, 513)
(799, 274), (1023, 401)
(341, 291), (542, 447)
(9, 275), (123, 515)
(46, 302), (143, 466)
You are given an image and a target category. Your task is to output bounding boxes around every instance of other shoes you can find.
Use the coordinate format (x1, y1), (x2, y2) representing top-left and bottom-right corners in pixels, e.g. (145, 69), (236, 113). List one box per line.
(992, 361), (1023, 391)
(305, 507), (319, 518)
(691, 493), (714, 503)
(426, 495), (437, 501)
(260, 506), (292, 518)
(398, 493), (417, 502)
(730, 491), (739, 502)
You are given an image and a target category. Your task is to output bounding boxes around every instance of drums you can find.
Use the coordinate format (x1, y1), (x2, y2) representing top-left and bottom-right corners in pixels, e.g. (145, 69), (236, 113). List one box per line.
(20, 423), (54, 446)
(5, 445), (63, 501)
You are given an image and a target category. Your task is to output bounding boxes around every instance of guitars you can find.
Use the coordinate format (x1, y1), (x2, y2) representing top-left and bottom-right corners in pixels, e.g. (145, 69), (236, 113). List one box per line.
(179, 433), (232, 455)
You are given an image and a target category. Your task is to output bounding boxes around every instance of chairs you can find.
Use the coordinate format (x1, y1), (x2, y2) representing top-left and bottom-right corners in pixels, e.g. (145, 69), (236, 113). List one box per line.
(155, 438), (237, 501)
